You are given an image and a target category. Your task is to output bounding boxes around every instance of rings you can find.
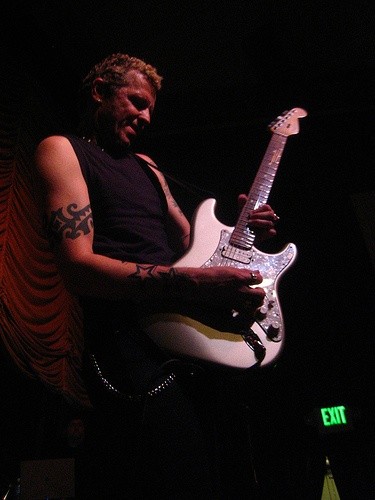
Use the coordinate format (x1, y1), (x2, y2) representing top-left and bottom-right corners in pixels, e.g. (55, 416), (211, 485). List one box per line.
(250, 271), (259, 283)
(274, 213), (280, 222)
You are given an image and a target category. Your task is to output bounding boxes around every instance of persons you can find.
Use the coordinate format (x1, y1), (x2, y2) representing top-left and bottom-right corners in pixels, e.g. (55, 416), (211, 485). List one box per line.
(35, 50), (281, 500)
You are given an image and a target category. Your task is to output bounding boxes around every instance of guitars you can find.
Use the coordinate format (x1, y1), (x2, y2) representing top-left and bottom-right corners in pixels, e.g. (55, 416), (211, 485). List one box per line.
(139, 107), (308, 368)
